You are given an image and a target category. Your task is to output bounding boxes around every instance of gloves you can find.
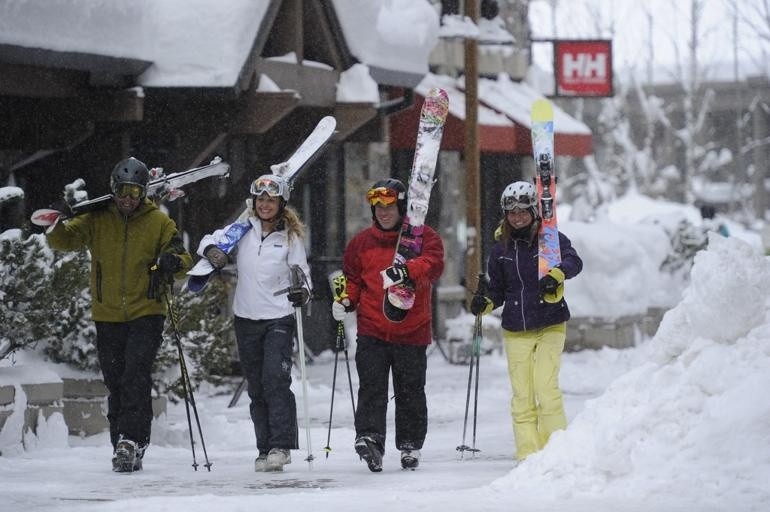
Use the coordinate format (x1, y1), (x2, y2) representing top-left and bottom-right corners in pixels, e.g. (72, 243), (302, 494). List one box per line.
(539, 275), (559, 299)
(152, 252), (177, 288)
(328, 296), (353, 320)
(471, 294), (489, 316)
(381, 265), (409, 288)
(285, 284), (310, 307)
(46, 197), (79, 220)
(203, 244), (230, 270)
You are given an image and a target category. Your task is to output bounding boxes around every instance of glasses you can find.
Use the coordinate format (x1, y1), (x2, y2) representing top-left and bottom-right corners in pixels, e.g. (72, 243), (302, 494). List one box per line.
(504, 194), (533, 211)
(367, 185), (397, 208)
(251, 179), (282, 197)
(115, 183), (144, 198)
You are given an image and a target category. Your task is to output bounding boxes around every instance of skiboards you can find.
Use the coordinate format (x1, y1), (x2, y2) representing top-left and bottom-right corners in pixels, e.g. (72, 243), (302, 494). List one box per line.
(30, 161), (230, 235)
(185, 116), (339, 292)
(530, 98), (563, 303)
(383, 89), (448, 324)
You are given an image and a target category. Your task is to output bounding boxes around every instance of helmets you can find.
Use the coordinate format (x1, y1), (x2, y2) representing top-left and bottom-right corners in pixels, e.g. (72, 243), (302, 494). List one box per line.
(248, 174), (294, 203)
(108, 156), (151, 197)
(500, 179), (542, 225)
(365, 179), (410, 221)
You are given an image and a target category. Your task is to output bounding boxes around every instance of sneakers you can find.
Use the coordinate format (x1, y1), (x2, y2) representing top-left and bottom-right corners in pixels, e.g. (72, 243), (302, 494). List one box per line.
(253, 446), (294, 474)
(111, 439), (144, 473)
(354, 436), (384, 471)
(399, 449), (422, 469)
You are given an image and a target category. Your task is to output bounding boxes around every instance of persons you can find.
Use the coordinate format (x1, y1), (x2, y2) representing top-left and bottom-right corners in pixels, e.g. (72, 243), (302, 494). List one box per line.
(333, 178), (444, 472)
(471, 181), (583, 461)
(47, 157), (194, 473)
(196, 174), (314, 471)
